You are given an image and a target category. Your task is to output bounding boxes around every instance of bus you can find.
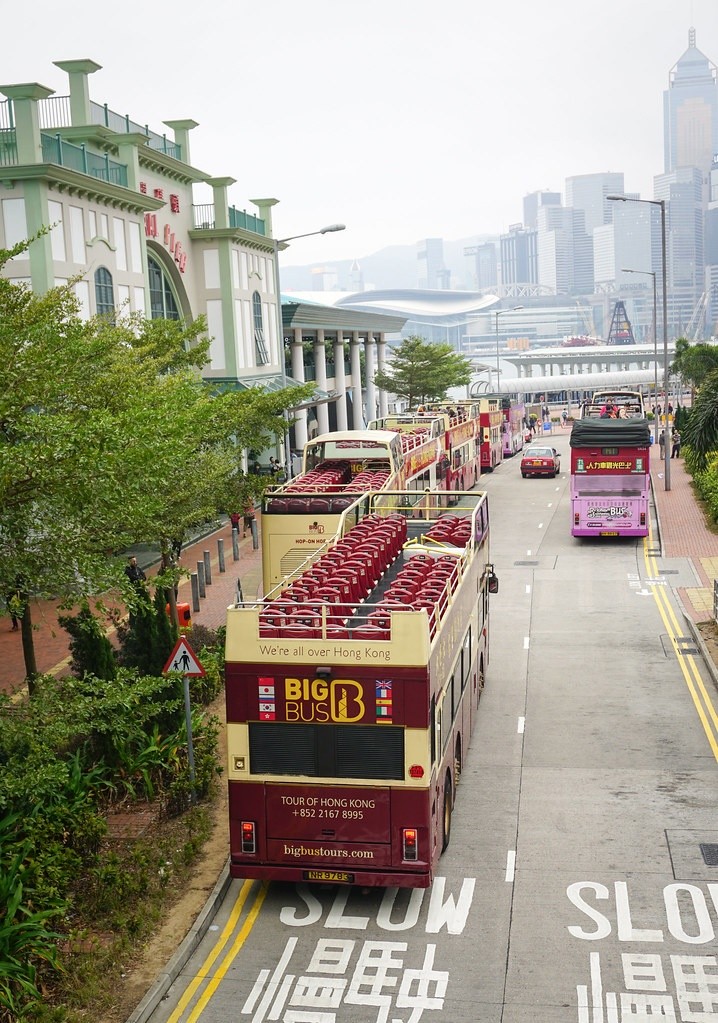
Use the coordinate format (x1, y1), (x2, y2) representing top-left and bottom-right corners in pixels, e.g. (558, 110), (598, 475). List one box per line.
(568, 391), (651, 537)
(263, 395), (525, 596)
(222, 487), (500, 889)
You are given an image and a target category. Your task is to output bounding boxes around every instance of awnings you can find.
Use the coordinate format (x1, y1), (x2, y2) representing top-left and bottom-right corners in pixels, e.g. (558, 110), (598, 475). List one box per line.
(208, 376), (342, 415)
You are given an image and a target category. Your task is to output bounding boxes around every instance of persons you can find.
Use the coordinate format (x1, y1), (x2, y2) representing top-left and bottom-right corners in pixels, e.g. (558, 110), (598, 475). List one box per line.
(652, 402), (680, 414)
(523, 417), (541, 434)
(229, 512), (240, 534)
(542, 407), (550, 422)
(157, 555), (180, 603)
(7, 591), (21, 631)
(126, 557), (146, 586)
(254, 464), (260, 474)
(284, 458), (293, 479)
(269, 457), (282, 476)
(418, 406), (466, 426)
(171, 536), (182, 559)
(599, 399), (618, 419)
(619, 404), (641, 419)
(244, 496), (255, 538)
(659, 430), (665, 460)
(562, 408), (567, 425)
(670, 430), (680, 458)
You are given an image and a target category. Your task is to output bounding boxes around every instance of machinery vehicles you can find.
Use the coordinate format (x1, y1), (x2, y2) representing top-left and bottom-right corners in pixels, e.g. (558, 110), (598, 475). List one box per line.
(683, 292), (711, 342)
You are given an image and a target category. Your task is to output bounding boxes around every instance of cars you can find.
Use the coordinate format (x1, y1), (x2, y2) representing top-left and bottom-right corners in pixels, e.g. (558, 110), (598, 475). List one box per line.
(520, 447), (561, 478)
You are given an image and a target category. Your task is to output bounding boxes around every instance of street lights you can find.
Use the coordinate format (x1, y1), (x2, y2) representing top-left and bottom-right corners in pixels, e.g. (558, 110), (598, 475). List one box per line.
(608, 194), (671, 493)
(620, 267), (659, 445)
(271, 225), (347, 470)
(495, 306), (525, 399)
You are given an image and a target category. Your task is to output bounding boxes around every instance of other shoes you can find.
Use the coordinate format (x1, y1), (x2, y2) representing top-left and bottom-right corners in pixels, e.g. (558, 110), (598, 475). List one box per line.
(243, 532), (246, 538)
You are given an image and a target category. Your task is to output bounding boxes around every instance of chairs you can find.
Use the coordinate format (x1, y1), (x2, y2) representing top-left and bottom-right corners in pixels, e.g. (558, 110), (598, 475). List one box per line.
(233, 404), (643, 638)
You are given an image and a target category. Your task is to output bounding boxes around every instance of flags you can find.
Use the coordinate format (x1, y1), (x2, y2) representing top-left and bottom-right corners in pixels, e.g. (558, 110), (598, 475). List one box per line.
(308, 410), (318, 434)
